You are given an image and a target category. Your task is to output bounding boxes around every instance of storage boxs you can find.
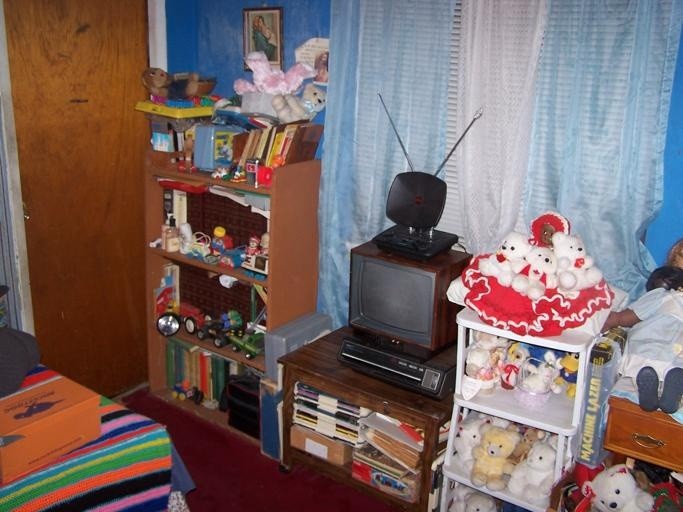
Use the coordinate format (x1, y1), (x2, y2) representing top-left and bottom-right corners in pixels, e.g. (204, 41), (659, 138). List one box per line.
(146, 114), (209, 134)
(289, 423), (353, 466)
(263, 312), (332, 383)
(0, 375), (102, 486)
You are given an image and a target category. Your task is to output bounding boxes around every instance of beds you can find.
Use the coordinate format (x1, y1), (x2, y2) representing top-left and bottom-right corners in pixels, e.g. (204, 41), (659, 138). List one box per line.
(0, 362), (196, 512)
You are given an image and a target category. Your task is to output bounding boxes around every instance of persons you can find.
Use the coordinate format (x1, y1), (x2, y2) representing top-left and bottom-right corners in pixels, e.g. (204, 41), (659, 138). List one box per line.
(600, 238), (683, 414)
(250, 15), (275, 62)
(258, 15), (277, 47)
(319, 50), (329, 83)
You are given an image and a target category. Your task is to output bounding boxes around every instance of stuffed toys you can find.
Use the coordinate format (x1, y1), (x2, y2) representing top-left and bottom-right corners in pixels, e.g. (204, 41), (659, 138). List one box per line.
(581, 464), (654, 512)
(446, 211), (616, 339)
(269, 82), (326, 123)
(448, 409), (571, 512)
(433, 207), (683, 512)
(140, 66), (199, 100)
(459, 330), (579, 408)
(233, 49), (319, 95)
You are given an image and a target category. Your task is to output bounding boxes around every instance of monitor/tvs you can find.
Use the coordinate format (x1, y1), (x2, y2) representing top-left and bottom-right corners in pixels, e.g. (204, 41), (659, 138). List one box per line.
(348, 233), (474, 361)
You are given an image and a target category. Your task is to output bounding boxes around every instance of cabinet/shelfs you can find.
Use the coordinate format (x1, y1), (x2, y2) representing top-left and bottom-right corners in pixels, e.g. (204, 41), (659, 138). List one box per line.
(144, 147), (321, 447)
(440, 286), (630, 512)
(602, 395), (683, 474)
(277, 326), (457, 512)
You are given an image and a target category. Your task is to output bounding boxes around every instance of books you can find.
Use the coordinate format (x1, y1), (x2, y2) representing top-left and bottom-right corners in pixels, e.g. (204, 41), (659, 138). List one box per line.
(229, 119), (325, 166)
(158, 337), (247, 413)
(160, 187), (188, 233)
(290, 379), (450, 482)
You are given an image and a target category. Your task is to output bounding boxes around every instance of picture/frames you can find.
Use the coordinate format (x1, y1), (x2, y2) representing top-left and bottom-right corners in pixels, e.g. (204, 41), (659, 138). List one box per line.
(242, 7), (283, 71)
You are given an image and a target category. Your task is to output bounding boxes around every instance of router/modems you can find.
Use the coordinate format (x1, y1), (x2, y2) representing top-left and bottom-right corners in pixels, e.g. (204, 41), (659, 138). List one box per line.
(385, 172), (447, 228)
